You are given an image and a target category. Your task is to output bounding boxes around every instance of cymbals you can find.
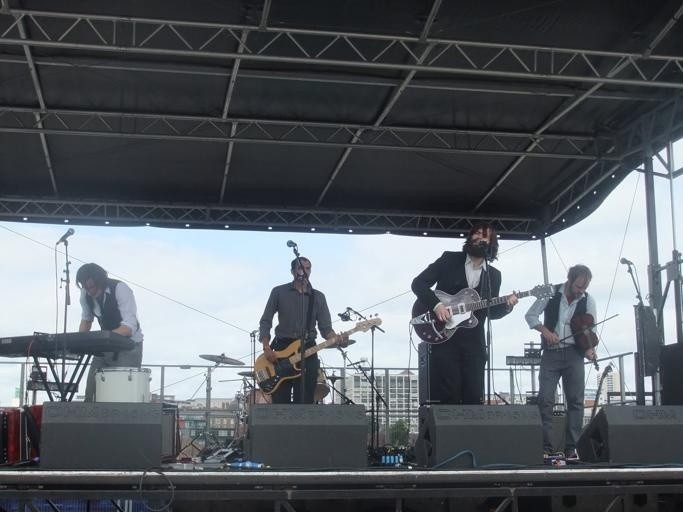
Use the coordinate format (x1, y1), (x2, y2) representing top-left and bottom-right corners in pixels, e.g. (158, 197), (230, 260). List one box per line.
(326, 376), (347, 379)
(199, 354), (246, 365)
(239, 372), (255, 377)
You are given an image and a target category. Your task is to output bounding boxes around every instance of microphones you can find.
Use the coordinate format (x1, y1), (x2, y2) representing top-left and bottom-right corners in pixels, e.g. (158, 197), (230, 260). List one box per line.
(57, 227), (74, 245)
(479, 237), (488, 260)
(287, 240), (299, 249)
(620, 258), (632, 264)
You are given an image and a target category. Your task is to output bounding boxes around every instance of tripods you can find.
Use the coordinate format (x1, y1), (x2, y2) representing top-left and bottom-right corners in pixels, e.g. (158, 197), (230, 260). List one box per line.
(178, 366), (253, 456)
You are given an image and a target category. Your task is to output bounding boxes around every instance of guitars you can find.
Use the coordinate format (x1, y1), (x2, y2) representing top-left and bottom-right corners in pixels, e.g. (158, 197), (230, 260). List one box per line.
(255, 313), (381, 395)
(413, 284), (558, 344)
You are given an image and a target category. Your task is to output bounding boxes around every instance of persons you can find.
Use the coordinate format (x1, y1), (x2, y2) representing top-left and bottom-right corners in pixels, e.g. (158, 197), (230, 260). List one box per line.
(411, 220), (517, 405)
(71, 262), (146, 403)
(525, 263), (601, 461)
(260, 257), (348, 403)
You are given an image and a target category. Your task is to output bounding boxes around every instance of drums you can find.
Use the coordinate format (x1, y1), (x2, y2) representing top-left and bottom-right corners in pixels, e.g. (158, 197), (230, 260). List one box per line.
(314, 369), (331, 401)
(95, 368), (152, 402)
(246, 388), (272, 414)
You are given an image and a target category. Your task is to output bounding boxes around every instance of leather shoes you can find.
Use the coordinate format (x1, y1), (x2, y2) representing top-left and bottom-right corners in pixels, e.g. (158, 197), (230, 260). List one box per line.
(565, 449), (577, 460)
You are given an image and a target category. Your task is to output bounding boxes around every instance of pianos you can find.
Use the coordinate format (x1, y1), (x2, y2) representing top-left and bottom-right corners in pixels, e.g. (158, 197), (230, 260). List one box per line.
(0, 331), (135, 357)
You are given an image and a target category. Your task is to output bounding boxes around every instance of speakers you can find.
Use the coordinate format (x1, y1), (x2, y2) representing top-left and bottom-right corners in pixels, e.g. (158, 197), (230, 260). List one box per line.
(577, 404), (682, 466)
(241, 403), (368, 470)
(36, 401), (176, 470)
(417, 402), (544, 467)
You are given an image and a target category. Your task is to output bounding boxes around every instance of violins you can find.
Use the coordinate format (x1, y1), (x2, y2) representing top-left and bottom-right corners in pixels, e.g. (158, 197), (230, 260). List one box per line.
(571, 314), (599, 370)
